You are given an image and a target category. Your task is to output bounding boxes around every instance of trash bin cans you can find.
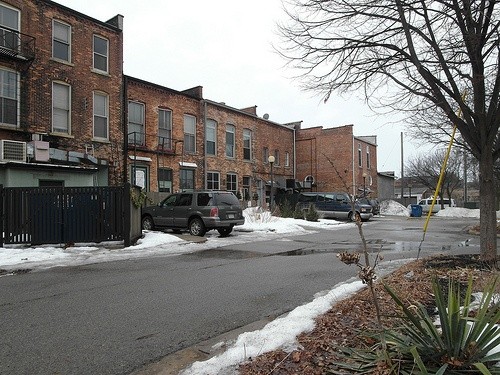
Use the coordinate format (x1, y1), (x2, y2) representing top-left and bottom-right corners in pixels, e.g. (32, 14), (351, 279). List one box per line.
(410, 205), (422, 217)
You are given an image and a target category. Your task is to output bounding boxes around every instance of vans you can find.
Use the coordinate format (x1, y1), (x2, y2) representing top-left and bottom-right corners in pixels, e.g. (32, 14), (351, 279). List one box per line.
(417, 198), (457, 213)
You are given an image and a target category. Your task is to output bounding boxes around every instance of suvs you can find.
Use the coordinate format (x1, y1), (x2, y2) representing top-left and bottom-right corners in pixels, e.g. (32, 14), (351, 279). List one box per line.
(292, 190), (373, 222)
(141, 188), (245, 238)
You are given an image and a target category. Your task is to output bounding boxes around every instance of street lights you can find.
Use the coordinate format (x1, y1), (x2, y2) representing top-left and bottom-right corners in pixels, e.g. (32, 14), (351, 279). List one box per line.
(268, 155), (276, 212)
(362, 172), (367, 197)
